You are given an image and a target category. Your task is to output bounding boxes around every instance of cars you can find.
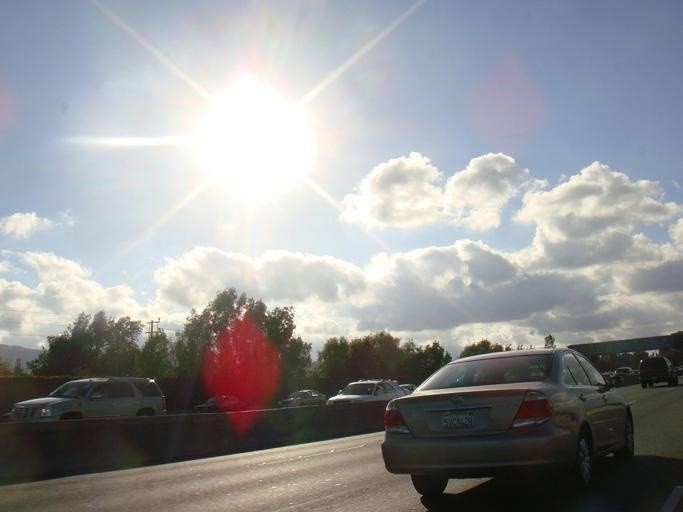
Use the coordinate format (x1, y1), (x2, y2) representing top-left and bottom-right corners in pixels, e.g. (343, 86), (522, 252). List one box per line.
(192, 394), (247, 413)
(327, 378), (417, 406)
(602, 355), (682, 389)
(277, 387), (326, 408)
(379, 346), (636, 499)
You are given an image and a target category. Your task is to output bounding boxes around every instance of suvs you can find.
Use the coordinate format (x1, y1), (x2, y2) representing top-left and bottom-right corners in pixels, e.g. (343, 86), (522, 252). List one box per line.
(8, 376), (168, 423)
